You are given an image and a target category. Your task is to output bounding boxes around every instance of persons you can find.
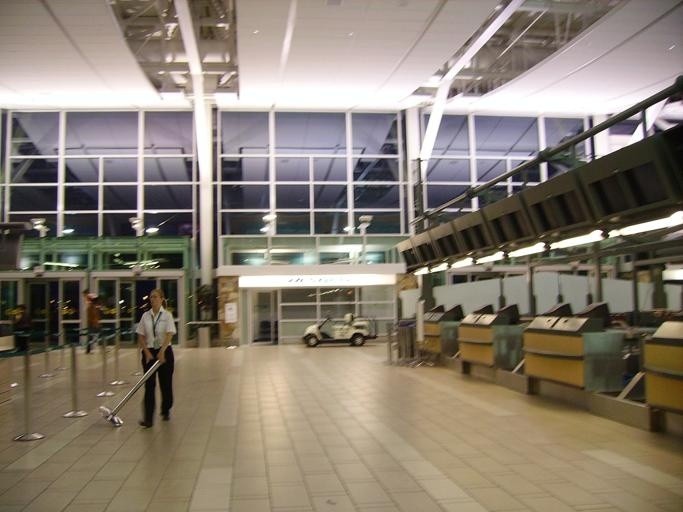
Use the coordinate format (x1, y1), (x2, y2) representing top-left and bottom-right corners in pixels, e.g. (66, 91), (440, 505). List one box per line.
(133, 286), (177, 428)
(79, 286), (100, 357)
(13, 304), (32, 354)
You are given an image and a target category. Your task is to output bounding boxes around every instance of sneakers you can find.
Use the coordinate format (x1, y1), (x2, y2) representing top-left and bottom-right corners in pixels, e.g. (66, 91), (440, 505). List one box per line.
(141, 421), (153, 426)
(163, 415), (170, 420)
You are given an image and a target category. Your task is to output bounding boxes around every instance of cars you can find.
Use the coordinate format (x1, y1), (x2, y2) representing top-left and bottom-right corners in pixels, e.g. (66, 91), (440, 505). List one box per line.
(302, 311), (378, 348)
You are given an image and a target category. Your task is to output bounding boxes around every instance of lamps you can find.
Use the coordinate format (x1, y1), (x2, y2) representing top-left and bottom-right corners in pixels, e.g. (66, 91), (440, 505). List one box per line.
(260, 213), (278, 222)
(144, 224), (158, 235)
(61, 225), (74, 234)
